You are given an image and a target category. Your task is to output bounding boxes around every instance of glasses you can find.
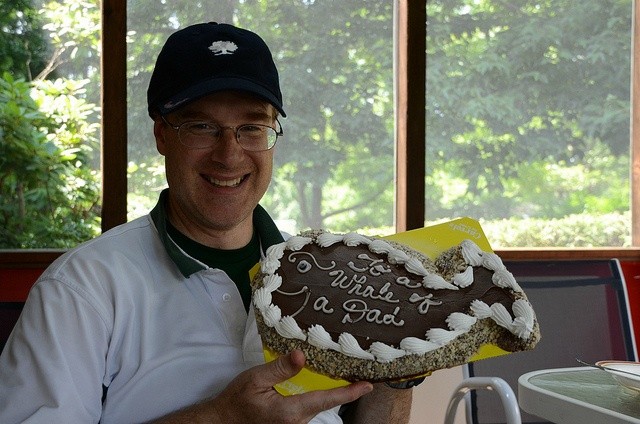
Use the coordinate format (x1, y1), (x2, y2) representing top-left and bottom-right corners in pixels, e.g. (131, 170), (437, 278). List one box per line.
(157, 116), (286, 151)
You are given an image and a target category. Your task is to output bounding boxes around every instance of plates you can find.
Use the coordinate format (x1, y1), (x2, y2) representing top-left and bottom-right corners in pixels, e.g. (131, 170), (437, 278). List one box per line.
(595, 360), (640, 392)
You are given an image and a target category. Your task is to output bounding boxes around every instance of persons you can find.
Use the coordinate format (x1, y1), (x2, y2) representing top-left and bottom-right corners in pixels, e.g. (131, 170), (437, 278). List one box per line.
(0, 21), (413, 423)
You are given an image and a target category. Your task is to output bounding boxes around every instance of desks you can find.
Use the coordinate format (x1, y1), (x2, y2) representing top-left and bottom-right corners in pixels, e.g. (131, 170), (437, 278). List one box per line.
(518, 368), (640, 424)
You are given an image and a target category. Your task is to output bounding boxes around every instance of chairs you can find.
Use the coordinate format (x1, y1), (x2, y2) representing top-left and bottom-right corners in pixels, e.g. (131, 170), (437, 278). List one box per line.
(444, 258), (639, 423)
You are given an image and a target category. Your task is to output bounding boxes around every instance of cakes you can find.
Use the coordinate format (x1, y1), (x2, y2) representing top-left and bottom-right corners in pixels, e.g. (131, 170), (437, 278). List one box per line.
(251, 229), (540, 383)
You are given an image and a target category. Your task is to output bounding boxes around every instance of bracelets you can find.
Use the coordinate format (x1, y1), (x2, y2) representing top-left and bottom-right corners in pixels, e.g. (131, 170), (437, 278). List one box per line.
(384, 377), (426, 389)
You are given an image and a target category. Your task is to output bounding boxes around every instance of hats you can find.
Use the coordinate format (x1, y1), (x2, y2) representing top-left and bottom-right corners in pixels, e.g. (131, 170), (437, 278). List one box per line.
(143, 20), (286, 118)
